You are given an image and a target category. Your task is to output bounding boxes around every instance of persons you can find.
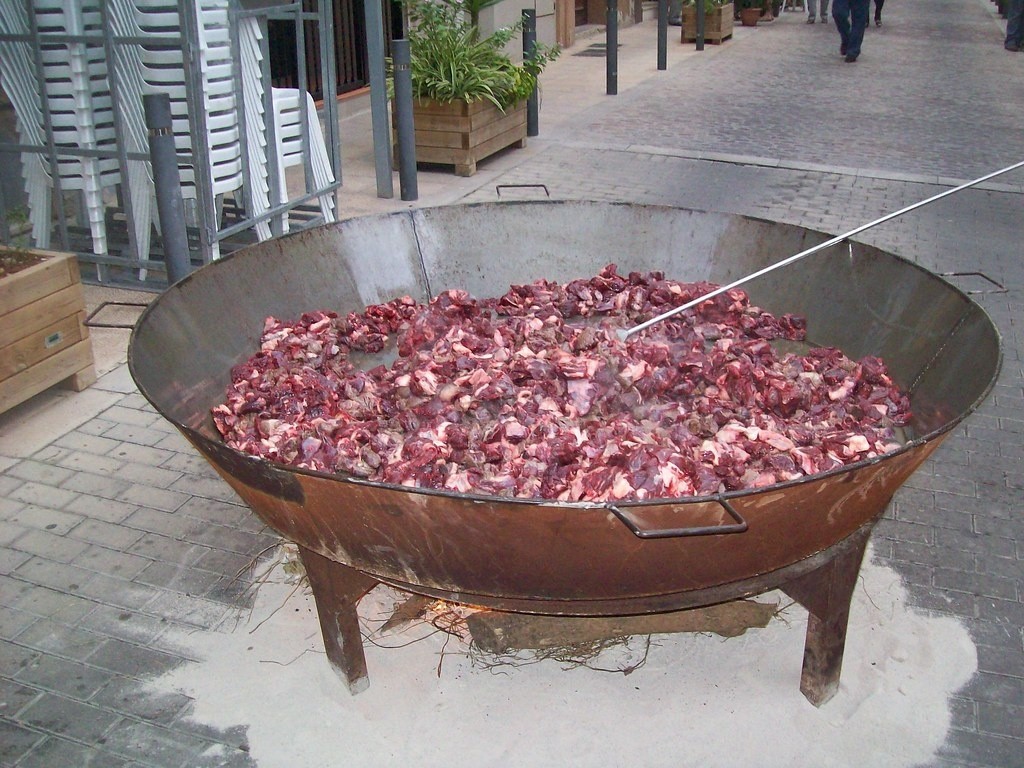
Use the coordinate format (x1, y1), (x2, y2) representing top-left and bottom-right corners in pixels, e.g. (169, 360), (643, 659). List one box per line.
(669, 0), (886, 63)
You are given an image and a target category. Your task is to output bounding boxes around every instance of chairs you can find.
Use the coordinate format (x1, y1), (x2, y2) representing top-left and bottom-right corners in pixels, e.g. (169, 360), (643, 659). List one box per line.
(0, 0), (346, 294)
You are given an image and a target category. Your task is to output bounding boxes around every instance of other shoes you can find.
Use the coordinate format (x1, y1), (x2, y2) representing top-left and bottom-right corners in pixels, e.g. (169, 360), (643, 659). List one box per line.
(1005, 43), (1023, 51)
(840, 41), (856, 62)
(806, 19), (828, 23)
(864, 19), (881, 26)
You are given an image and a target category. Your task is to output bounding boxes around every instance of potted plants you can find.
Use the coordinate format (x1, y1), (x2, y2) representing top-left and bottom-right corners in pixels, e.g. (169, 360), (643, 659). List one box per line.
(680, 0), (736, 46)
(380, 1), (563, 178)
(739, 0), (765, 27)
(0, 201), (97, 416)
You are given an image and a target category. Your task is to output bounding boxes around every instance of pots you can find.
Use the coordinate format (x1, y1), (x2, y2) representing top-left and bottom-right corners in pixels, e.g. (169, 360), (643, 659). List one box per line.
(80, 179), (1007, 608)
